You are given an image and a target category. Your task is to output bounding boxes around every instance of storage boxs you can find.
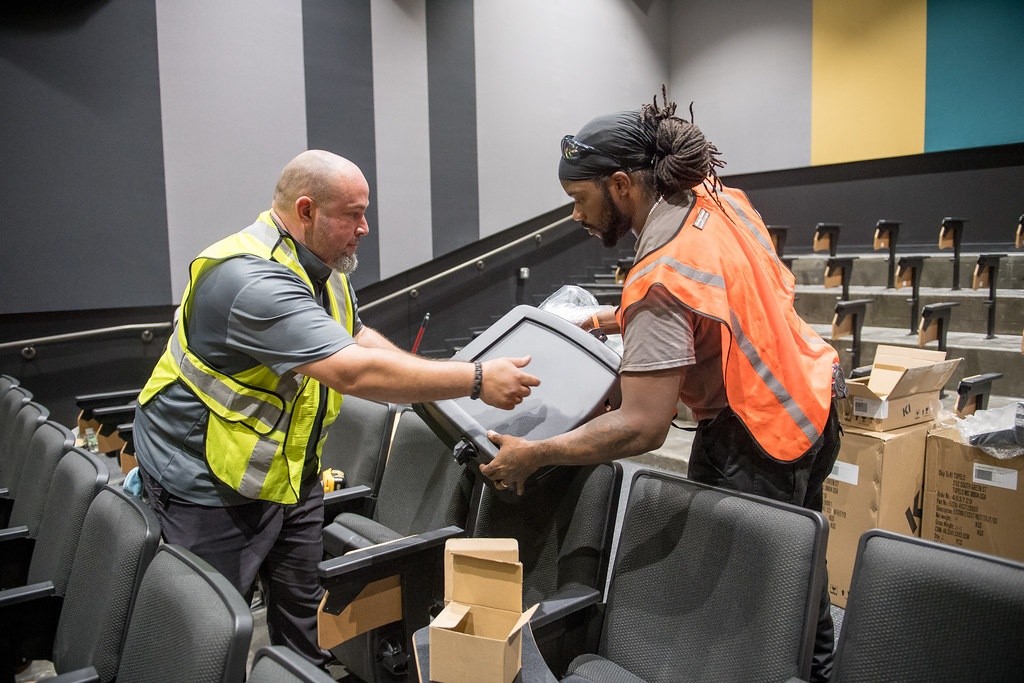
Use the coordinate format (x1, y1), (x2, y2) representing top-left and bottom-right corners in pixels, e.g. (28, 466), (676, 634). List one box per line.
(921, 426), (1024, 562)
(429, 552), (540, 683)
(839, 343), (965, 433)
(823, 419), (958, 609)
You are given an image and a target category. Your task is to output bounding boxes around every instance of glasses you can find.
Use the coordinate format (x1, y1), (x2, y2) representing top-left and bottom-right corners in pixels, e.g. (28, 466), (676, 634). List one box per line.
(560, 134), (633, 174)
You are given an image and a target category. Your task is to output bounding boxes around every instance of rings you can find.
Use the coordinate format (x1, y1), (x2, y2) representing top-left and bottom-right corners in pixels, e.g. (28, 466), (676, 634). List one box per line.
(500, 481), (510, 487)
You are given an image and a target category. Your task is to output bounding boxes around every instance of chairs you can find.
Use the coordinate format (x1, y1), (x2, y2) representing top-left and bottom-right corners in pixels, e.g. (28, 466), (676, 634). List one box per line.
(0, 420), (76, 537)
(830, 527), (1024, 683)
(33, 544), (255, 683)
(318, 459), (624, 683)
(0, 483), (161, 683)
(320, 395), (398, 497)
(0, 374), (20, 403)
(528, 470), (829, 683)
(0, 446), (110, 594)
(0, 401), (50, 498)
(321, 407), (485, 557)
(0, 387), (33, 450)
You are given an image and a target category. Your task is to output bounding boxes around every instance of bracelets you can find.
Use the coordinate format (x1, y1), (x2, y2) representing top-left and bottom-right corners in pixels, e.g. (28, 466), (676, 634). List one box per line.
(591, 312), (599, 328)
(470, 361), (482, 401)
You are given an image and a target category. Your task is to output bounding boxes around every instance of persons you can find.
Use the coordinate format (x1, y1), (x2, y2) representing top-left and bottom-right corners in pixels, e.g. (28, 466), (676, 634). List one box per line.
(133, 149), (541, 676)
(479, 82), (845, 683)
